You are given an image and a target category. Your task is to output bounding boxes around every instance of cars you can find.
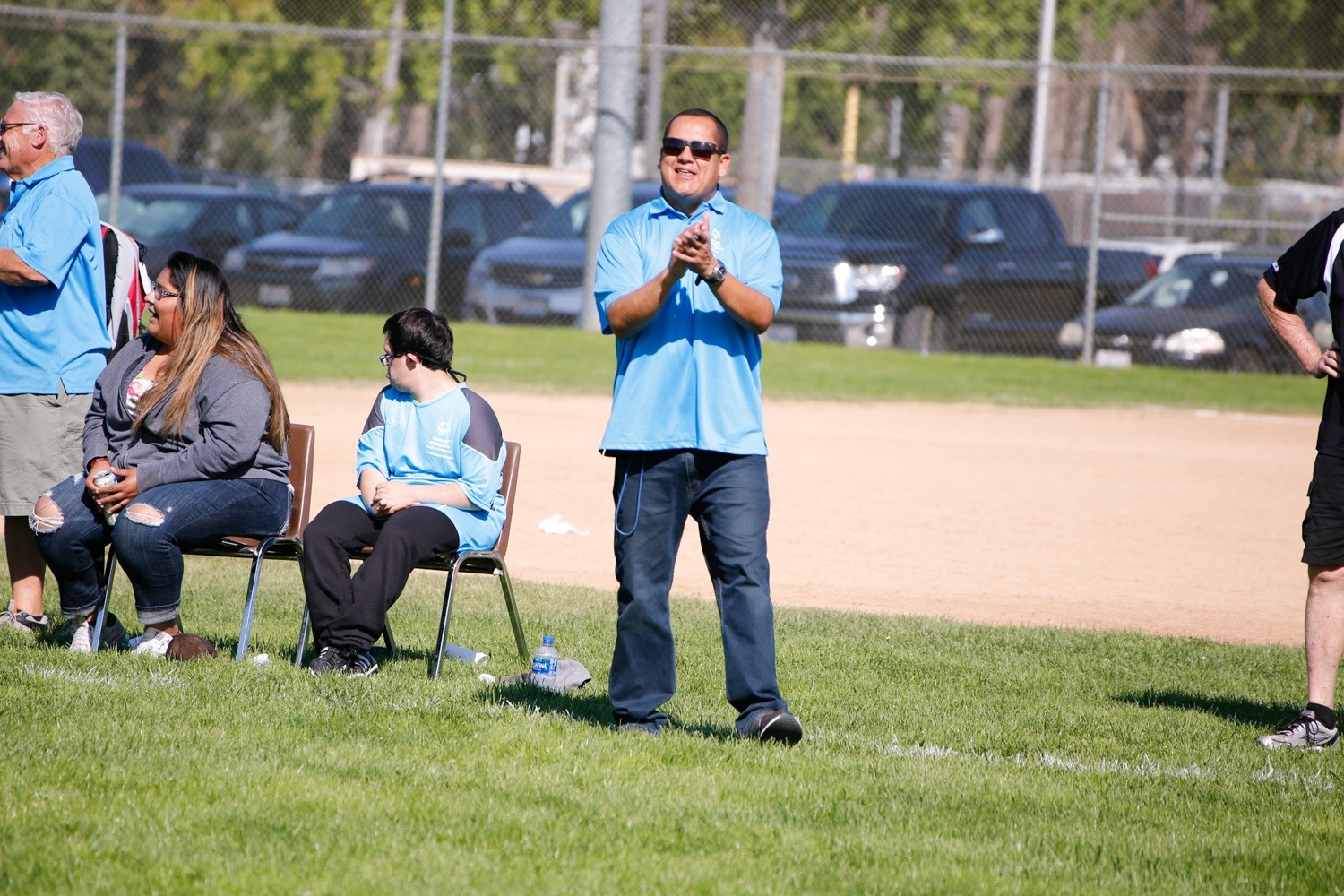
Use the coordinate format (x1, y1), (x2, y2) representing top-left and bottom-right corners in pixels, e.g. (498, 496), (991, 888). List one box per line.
(1055, 253), (1338, 378)
(95, 181), (317, 301)
(460, 178), (807, 339)
(1076, 235), (1243, 294)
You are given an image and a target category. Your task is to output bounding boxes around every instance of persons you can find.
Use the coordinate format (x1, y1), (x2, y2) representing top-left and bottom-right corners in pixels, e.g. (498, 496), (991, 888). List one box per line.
(0, 91), (112, 649)
(1250, 207), (1344, 752)
(27, 250), (296, 658)
(594, 108), (803, 747)
(303, 307), (507, 679)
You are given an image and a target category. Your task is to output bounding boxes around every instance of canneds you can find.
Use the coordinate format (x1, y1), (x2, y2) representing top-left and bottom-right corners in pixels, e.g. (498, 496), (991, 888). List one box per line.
(93, 470), (123, 525)
(444, 643), (486, 667)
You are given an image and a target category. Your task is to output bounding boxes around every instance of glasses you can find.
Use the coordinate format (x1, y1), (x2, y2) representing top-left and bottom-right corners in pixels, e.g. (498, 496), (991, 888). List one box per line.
(379, 351), (409, 366)
(151, 282), (183, 301)
(0, 120), (48, 137)
(662, 138), (725, 162)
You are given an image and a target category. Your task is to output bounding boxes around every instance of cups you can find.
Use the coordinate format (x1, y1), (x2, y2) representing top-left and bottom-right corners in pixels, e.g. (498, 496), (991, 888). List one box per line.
(446, 642), (488, 666)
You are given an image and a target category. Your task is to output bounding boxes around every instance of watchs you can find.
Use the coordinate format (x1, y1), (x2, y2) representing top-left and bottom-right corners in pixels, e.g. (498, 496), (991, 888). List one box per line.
(695, 259), (727, 287)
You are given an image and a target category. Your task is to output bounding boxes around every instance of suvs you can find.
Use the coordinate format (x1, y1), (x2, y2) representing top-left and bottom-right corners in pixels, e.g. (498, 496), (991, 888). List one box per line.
(0, 141), (183, 234)
(217, 169), (582, 322)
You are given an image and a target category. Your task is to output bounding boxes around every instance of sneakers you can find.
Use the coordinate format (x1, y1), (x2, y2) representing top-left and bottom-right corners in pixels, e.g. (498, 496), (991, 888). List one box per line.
(308, 646), (345, 678)
(131, 632), (181, 659)
(98, 612), (130, 651)
(0, 600), (51, 637)
(69, 624), (95, 654)
(758, 711), (803, 746)
(339, 651), (378, 679)
(129, 627), (161, 649)
(1257, 709), (1340, 752)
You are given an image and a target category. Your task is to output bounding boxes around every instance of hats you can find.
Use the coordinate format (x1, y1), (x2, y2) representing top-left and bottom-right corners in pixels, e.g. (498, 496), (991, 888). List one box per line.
(508, 659), (592, 694)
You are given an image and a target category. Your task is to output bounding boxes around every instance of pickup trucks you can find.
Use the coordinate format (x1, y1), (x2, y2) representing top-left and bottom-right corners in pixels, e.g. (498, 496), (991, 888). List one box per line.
(768, 180), (1165, 353)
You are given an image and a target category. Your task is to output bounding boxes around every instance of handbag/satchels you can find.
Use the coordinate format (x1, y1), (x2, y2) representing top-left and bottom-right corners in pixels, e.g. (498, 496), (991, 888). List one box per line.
(100, 221), (152, 365)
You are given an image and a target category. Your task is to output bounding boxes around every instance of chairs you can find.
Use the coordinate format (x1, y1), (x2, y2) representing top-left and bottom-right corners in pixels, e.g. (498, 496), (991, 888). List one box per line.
(295, 440), (531, 681)
(90, 424), (315, 667)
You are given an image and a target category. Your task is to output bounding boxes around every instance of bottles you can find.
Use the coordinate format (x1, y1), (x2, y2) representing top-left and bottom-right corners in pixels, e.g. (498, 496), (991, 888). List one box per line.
(531, 635), (558, 689)
